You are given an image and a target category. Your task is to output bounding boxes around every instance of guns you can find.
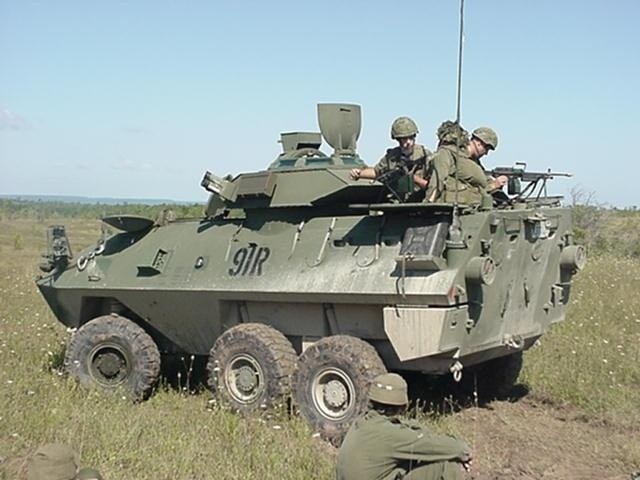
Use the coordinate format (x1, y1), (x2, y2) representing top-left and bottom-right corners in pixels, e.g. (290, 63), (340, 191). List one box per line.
(378, 155), (428, 189)
(486, 164), (572, 200)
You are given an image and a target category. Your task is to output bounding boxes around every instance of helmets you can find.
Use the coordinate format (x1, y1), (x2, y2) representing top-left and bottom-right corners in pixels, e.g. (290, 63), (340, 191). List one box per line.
(367, 372), (409, 406)
(391, 117), (419, 139)
(472, 127), (497, 150)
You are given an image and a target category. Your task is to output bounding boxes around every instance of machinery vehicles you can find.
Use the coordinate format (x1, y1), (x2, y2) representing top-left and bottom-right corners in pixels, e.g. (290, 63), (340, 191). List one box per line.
(37, 0), (586, 447)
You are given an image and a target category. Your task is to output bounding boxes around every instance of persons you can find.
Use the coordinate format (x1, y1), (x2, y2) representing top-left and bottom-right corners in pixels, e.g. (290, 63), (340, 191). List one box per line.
(463, 126), (508, 201)
(335, 373), (474, 480)
(27, 443), (102, 480)
(419, 121), (488, 208)
(349, 117), (439, 198)
